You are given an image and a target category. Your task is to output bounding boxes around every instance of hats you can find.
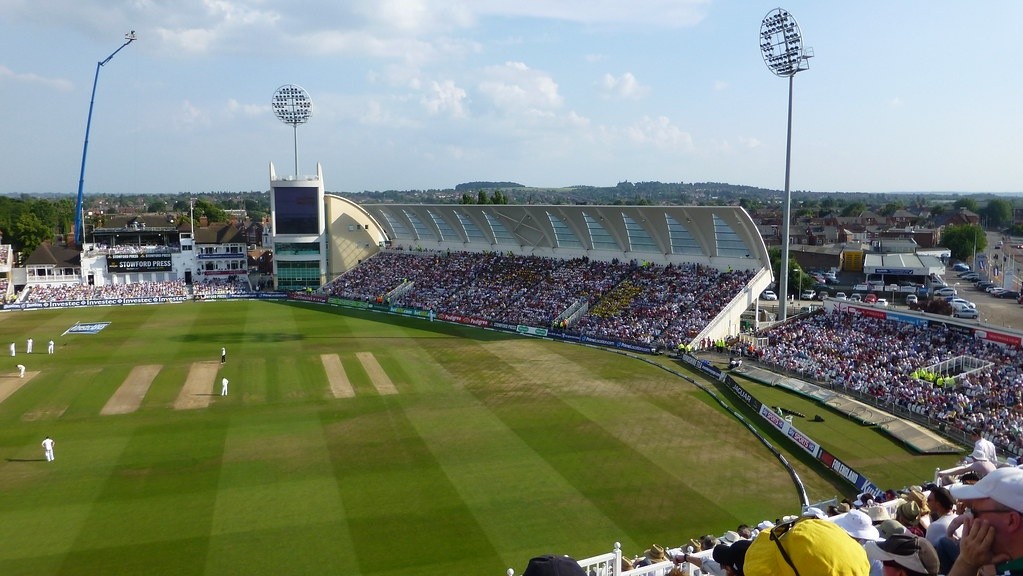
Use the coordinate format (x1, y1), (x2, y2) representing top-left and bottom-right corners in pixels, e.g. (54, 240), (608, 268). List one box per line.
(521, 553), (588, 576)
(950, 467), (1023, 515)
(834, 503), (851, 514)
(782, 515), (800, 523)
(833, 508), (887, 543)
(1003, 457), (1018, 467)
(742, 518), (871, 576)
(663, 568), (690, 576)
(758, 520), (774, 530)
(875, 519), (904, 539)
(901, 489), (931, 516)
(643, 544), (670, 563)
(611, 556), (635, 570)
(681, 538), (703, 555)
(968, 450), (988, 461)
(664, 548), (685, 559)
(865, 534), (940, 576)
(867, 507), (892, 521)
(802, 508), (828, 519)
(896, 500), (921, 526)
(718, 530), (741, 547)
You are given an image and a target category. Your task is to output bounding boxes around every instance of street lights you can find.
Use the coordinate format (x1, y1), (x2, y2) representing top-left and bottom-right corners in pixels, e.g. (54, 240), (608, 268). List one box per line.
(759, 7), (815, 320)
(793, 268), (802, 305)
(272, 83), (313, 179)
(952, 282), (960, 317)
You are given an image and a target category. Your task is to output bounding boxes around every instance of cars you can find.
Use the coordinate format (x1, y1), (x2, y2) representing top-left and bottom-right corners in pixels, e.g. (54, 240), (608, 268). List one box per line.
(904, 294), (919, 305)
(761, 290), (777, 301)
(863, 293), (878, 305)
(949, 262), (1023, 318)
(876, 298), (889, 308)
(996, 240), (1004, 247)
(1016, 243), (1023, 249)
(835, 291), (847, 302)
(817, 291), (829, 302)
(849, 293), (862, 303)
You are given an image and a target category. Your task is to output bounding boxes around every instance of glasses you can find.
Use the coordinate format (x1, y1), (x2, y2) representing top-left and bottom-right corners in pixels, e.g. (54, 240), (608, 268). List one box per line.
(964, 507), (1010, 519)
(768, 514), (819, 575)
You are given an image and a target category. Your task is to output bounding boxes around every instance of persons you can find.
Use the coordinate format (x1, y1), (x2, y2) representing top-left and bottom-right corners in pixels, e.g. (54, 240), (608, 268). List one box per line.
(16, 364), (25, 378)
(729, 306), (1023, 448)
(522, 428), (1023, 576)
(28, 278), (187, 302)
(48, 340), (54, 354)
(0, 279), (8, 298)
(306, 244), (758, 353)
(27, 338), (33, 353)
(0, 246), (8, 265)
(42, 436), (55, 461)
(193, 279), (246, 294)
(96, 243), (180, 253)
(222, 377), (228, 396)
(10, 342), (15, 356)
(256, 278), (273, 292)
(221, 348), (226, 364)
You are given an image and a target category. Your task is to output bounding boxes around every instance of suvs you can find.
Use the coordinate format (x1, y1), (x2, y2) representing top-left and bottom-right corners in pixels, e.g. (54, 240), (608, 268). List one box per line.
(933, 287), (958, 298)
(801, 289), (817, 301)
